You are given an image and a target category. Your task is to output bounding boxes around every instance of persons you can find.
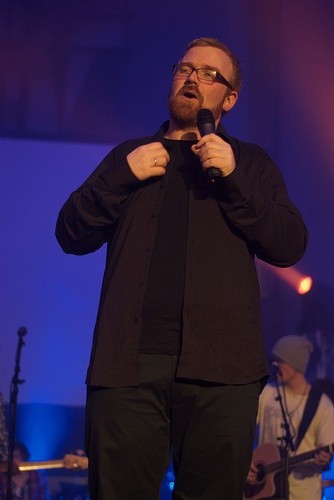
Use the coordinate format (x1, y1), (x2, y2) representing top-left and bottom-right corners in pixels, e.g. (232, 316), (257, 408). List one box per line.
(55, 39), (308, 500)
(245, 335), (334, 500)
(11, 443), (38, 500)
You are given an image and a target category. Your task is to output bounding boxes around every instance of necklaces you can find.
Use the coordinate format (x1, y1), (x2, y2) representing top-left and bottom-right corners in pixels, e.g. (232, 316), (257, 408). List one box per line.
(283, 382), (308, 418)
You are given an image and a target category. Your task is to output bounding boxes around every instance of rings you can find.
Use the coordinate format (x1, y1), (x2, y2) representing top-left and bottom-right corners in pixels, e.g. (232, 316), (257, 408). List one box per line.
(154, 158), (157, 165)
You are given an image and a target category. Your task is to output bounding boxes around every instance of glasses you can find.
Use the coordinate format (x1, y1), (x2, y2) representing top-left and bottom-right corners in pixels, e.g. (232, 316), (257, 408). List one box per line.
(173, 64), (234, 91)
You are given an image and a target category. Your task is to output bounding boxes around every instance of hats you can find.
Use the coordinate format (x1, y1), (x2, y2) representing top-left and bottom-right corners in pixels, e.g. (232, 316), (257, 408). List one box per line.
(271, 336), (313, 373)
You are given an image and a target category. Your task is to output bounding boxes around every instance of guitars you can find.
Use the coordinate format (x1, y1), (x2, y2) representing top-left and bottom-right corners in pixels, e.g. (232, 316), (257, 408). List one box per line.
(0, 448), (89, 473)
(240, 443), (334, 500)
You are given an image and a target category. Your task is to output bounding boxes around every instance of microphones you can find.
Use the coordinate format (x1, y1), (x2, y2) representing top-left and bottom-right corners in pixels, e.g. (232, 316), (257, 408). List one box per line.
(197, 109), (222, 182)
(271, 362), (278, 375)
